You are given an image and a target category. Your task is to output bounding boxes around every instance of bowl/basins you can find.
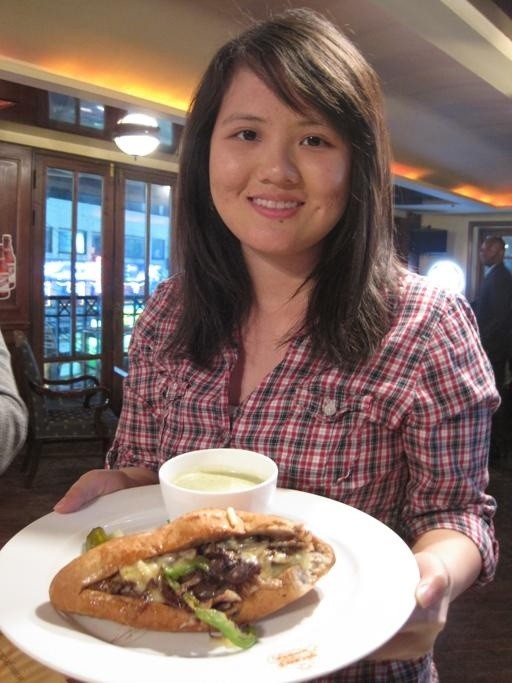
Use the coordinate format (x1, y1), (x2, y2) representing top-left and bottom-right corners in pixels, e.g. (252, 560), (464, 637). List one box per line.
(157, 448), (280, 523)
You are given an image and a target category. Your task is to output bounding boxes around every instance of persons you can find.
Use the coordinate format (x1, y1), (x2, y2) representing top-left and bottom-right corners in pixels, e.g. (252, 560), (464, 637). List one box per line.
(1, 329), (30, 477)
(53, 9), (503, 683)
(475, 237), (512, 468)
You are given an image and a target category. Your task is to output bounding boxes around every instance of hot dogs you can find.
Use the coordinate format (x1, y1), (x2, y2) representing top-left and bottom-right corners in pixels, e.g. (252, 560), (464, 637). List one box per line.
(48, 508), (335, 633)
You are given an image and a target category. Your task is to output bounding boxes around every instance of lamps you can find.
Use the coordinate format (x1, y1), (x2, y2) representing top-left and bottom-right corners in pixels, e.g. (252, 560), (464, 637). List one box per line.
(111, 113), (161, 161)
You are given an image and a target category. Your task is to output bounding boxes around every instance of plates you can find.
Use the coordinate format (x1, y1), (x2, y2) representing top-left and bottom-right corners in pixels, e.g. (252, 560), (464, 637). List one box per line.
(0, 480), (422, 683)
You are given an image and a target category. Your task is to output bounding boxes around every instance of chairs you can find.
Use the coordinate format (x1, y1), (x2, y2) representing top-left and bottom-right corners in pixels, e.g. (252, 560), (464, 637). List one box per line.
(12, 330), (119, 488)
